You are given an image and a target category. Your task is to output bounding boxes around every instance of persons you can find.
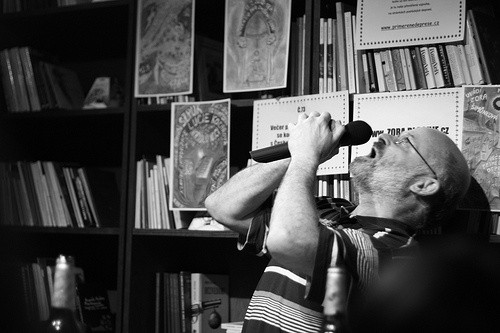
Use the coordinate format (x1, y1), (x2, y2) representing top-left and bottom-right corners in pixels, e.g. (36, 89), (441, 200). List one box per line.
(204, 111), (470, 333)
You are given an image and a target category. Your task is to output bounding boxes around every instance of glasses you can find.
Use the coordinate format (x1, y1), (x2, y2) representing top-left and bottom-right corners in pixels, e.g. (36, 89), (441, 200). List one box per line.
(394, 135), (438, 180)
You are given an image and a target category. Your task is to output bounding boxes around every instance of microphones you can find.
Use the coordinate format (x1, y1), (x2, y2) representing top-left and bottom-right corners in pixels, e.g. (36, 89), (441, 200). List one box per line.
(249, 120), (372, 163)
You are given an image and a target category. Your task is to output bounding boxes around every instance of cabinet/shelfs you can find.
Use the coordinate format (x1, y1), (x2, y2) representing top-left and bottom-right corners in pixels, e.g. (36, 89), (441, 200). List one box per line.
(0, 0), (500, 333)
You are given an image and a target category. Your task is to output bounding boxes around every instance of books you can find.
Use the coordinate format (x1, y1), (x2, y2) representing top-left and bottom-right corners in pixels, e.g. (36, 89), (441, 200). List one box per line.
(11, 264), (118, 333)
(297, 15), (304, 96)
(0, 162), (99, 226)
(135, 156), (186, 229)
(1, 47), (71, 112)
(155, 272), (222, 333)
(315, 175), (350, 201)
(318, 2), (492, 94)
(145, 95), (195, 103)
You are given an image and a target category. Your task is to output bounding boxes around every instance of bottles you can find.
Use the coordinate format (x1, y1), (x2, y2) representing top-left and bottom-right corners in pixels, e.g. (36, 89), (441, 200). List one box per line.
(37, 254), (84, 333)
